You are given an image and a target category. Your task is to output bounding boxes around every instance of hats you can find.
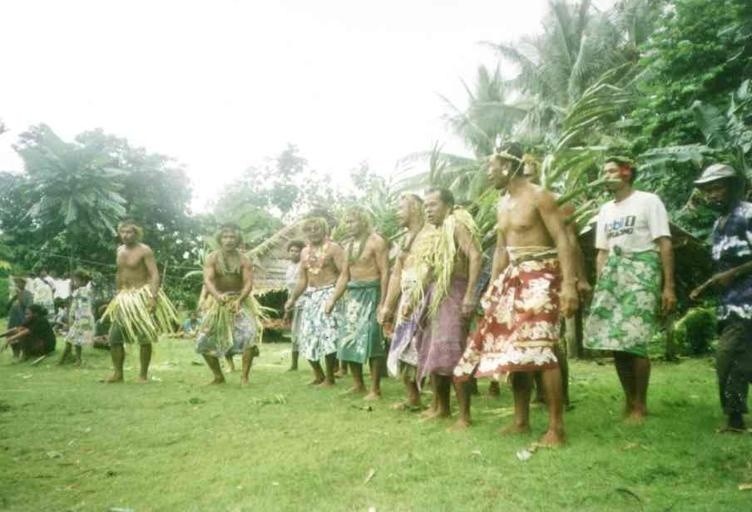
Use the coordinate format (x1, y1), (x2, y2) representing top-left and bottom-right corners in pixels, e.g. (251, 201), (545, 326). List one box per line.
(693, 164), (736, 184)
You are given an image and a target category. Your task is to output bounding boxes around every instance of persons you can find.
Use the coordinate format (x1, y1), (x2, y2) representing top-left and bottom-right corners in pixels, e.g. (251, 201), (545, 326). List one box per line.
(690, 163), (752, 434)
(375, 193), (436, 412)
(223, 241), (260, 374)
(283, 204), (390, 401)
(523, 153), (594, 414)
(98, 218), (162, 384)
(0, 262), (96, 367)
(194, 221), (263, 394)
(482, 143), (579, 452)
(402, 186), (501, 433)
(581, 154), (678, 420)
